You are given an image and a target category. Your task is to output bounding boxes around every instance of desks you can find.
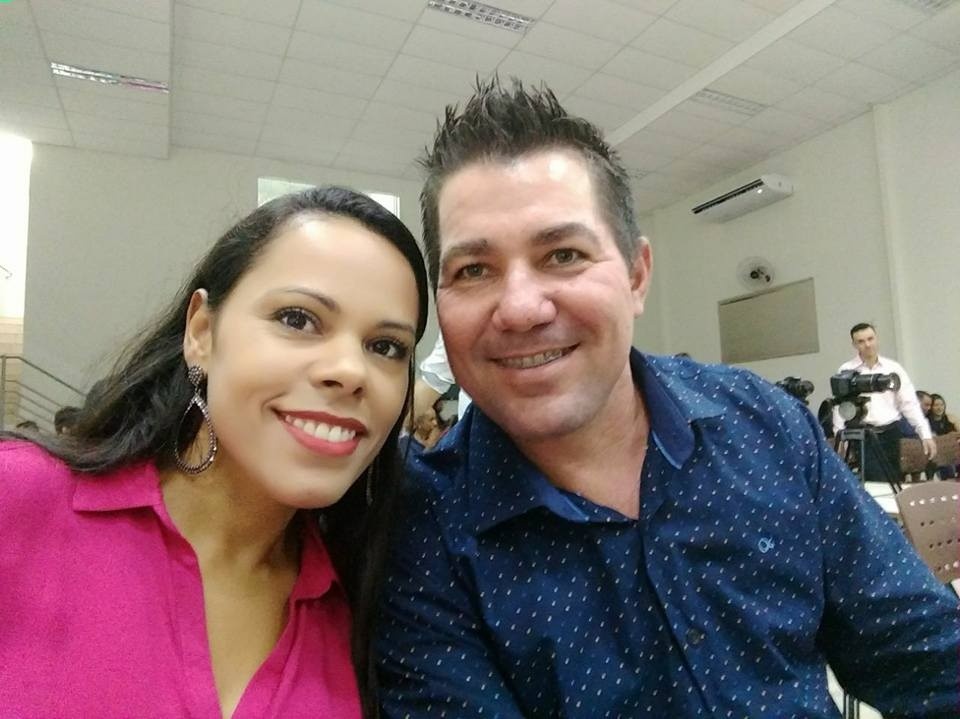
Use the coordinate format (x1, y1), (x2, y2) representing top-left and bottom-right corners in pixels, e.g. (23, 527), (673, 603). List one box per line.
(864, 479), (918, 519)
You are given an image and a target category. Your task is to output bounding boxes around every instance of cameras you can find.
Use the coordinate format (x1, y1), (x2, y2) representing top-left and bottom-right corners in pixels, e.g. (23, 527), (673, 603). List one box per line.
(830, 370), (901, 397)
(775, 376), (815, 400)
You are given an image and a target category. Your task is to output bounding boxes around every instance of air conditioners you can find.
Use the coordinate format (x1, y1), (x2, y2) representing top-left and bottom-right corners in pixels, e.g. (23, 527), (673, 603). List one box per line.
(688, 174), (793, 224)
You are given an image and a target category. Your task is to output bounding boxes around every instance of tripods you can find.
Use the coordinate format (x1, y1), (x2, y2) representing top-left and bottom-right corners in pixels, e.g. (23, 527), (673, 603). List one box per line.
(828, 396), (902, 719)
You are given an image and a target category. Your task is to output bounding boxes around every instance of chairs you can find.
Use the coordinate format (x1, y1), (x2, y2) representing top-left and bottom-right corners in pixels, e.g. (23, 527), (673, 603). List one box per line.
(893, 431), (960, 596)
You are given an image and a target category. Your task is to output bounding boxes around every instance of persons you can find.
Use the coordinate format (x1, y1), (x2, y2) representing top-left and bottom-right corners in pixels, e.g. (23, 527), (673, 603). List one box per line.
(0, 187), (429, 719)
(407, 323), (960, 487)
(356, 67), (960, 719)
